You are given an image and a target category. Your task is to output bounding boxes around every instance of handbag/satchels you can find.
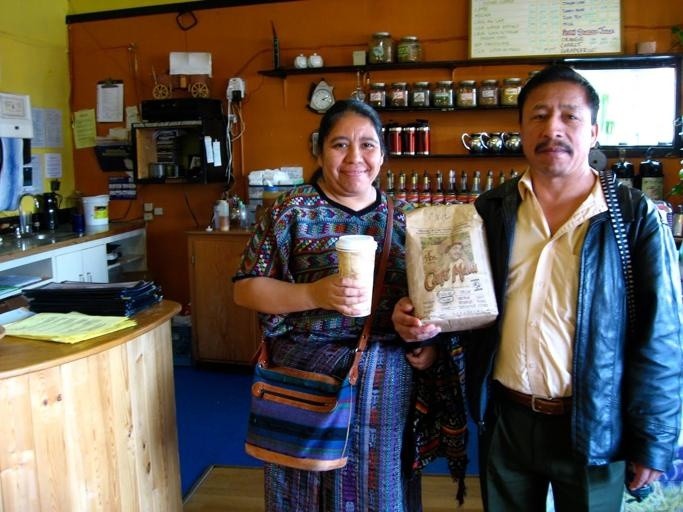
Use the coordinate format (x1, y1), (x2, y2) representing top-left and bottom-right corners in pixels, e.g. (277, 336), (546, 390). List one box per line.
(245, 364), (356, 470)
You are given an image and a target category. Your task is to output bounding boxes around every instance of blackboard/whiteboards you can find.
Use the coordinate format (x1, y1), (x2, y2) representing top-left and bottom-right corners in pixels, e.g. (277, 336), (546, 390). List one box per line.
(467, 0), (626, 62)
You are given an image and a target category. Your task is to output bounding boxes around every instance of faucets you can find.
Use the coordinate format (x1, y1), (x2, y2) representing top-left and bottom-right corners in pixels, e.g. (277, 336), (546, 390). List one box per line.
(18, 193), (39, 234)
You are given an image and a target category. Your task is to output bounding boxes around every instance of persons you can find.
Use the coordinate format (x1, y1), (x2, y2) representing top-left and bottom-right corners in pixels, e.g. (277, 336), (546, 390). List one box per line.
(230, 100), (438, 511)
(389, 63), (682, 512)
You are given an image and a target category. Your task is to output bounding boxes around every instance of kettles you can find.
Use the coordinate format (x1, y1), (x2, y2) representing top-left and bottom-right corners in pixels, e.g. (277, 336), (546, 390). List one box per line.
(33, 191), (63, 234)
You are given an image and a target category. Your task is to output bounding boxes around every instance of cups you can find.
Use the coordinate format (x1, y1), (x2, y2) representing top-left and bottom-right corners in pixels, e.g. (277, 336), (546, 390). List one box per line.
(334, 234), (377, 319)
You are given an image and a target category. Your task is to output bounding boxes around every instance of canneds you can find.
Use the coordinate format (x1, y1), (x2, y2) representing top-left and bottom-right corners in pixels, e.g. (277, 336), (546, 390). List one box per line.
(395, 36), (423, 63)
(368, 70), (540, 107)
(369, 31), (394, 63)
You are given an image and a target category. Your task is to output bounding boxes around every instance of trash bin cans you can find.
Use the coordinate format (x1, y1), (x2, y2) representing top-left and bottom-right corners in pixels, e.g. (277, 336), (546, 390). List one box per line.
(171, 316), (193, 366)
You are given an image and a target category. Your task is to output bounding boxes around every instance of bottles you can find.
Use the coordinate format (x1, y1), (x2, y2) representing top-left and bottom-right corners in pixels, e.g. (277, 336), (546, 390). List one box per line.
(381, 119), (432, 157)
(368, 70), (541, 109)
(214, 196), (244, 232)
(373, 168), (524, 208)
(611, 147), (665, 205)
(367, 30), (423, 63)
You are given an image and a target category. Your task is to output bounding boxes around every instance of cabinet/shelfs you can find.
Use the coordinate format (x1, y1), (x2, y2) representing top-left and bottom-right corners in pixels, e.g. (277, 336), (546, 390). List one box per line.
(106, 227), (149, 284)
(255, 59), (683, 158)
(185, 235), (255, 363)
(54, 244), (107, 283)
(0, 250), (53, 303)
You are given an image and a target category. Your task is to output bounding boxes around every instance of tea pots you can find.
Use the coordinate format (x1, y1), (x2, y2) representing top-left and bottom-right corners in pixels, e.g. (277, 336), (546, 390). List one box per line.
(460, 130), (522, 154)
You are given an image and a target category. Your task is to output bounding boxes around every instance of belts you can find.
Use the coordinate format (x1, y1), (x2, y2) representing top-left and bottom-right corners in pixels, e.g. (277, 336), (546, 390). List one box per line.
(495, 381), (566, 415)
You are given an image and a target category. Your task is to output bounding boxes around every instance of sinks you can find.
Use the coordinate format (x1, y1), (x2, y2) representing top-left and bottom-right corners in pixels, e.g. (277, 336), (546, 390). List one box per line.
(10, 231), (74, 245)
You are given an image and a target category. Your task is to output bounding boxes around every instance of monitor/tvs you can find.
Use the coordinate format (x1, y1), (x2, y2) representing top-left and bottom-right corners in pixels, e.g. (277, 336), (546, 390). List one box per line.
(564, 54), (683, 158)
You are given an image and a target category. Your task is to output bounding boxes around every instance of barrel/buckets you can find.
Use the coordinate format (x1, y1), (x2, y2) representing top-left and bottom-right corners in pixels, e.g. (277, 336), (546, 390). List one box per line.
(81, 194), (110, 225)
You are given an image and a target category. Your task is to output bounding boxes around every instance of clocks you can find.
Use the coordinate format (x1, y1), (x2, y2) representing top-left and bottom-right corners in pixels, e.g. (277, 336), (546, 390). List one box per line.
(302, 76), (338, 116)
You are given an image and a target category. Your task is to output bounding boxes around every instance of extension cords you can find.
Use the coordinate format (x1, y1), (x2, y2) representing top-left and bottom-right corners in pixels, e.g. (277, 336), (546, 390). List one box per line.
(228, 78), (243, 99)
